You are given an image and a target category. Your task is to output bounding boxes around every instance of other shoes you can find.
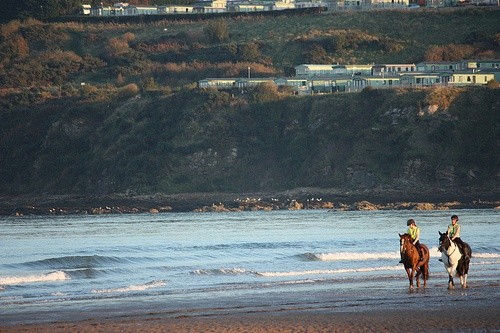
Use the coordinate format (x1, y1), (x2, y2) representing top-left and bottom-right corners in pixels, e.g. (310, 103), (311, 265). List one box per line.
(399, 260), (403, 263)
(464, 255), (469, 260)
(420, 256), (423, 261)
(438, 258), (442, 262)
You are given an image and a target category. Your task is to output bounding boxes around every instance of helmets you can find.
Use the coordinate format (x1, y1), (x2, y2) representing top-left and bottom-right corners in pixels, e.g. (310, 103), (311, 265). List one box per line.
(451, 215), (458, 221)
(407, 219), (414, 226)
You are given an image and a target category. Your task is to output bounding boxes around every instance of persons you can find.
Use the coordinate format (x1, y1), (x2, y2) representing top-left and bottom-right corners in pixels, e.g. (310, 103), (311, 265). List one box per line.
(439, 215), (461, 263)
(398, 219), (424, 263)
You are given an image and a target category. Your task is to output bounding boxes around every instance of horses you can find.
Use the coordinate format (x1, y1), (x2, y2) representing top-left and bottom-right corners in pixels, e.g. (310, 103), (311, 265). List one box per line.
(399, 233), (430, 289)
(438, 231), (472, 290)
